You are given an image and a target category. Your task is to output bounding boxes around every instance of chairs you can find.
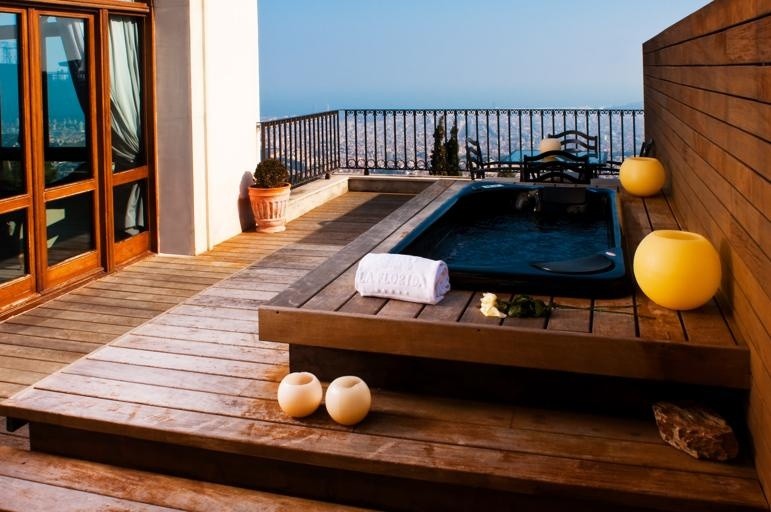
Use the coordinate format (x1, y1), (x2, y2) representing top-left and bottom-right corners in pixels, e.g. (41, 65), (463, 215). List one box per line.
(522, 149), (590, 184)
(594, 136), (654, 179)
(549, 131), (598, 177)
(465, 137), (521, 180)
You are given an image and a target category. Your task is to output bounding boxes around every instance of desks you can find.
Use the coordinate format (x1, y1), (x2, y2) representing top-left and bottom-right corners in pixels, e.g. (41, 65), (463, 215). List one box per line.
(499, 149), (608, 175)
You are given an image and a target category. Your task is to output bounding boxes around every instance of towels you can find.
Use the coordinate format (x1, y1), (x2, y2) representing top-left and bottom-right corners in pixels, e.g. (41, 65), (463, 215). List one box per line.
(355, 251), (450, 306)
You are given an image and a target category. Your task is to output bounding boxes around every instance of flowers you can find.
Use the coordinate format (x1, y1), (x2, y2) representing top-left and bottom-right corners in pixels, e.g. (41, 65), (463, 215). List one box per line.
(478, 289), (658, 319)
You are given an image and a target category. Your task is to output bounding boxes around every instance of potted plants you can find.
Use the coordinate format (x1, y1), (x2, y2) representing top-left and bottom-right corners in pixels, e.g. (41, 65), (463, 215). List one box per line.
(248, 159), (291, 234)
